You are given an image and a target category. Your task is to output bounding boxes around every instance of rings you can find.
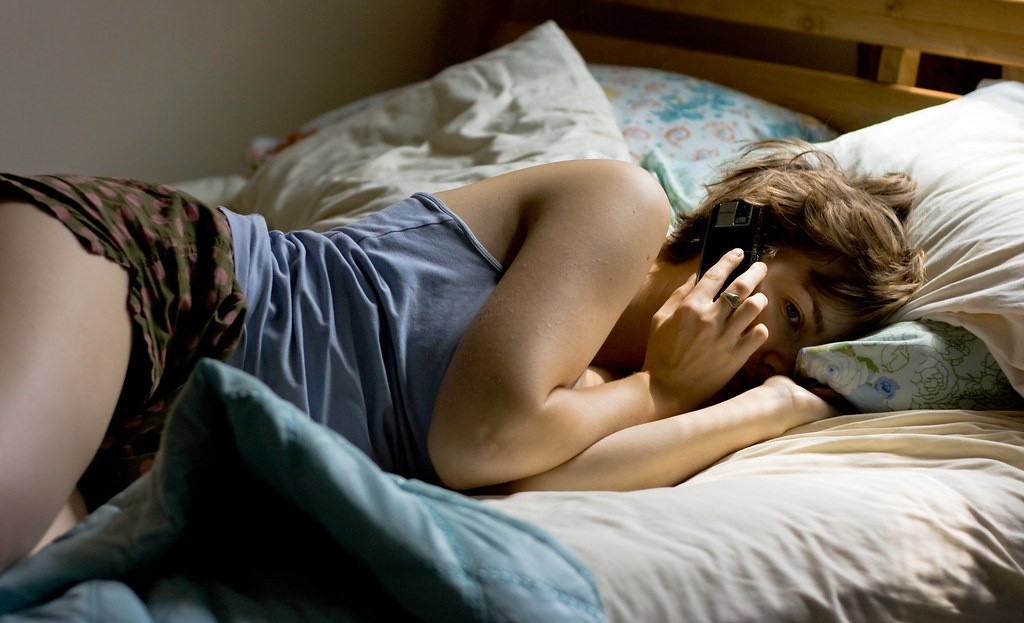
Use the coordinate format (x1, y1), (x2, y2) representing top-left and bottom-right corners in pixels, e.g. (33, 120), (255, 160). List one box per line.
(720, 291), (741, 307)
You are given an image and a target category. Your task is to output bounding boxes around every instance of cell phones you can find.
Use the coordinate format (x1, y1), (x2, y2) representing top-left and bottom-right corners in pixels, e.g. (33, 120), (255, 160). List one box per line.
(695, 200), (762, 303)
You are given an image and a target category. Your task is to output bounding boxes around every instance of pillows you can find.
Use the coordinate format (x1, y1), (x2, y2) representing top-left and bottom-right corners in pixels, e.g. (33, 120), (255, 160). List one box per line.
(242, 63), (1024, 413)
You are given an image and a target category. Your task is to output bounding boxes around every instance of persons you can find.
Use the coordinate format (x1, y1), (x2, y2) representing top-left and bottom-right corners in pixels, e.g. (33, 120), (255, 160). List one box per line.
(0, 137), (924, 574)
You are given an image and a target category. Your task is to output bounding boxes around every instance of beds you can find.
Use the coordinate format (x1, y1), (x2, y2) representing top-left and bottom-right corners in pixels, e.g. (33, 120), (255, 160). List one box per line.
(0, 0), (1024, 622)
